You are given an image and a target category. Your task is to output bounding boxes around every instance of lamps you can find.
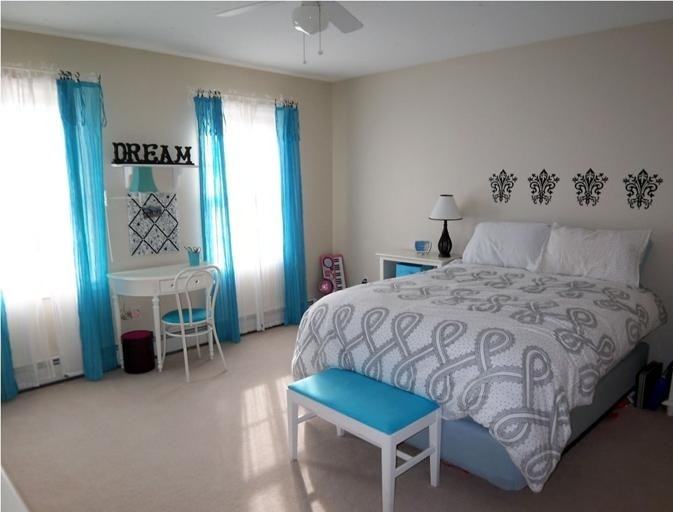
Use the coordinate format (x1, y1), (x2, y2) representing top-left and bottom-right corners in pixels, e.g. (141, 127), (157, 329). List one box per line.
(105, 164), (158, 211)
(291, 5), (330, 34)
(429, 194), (462, 258)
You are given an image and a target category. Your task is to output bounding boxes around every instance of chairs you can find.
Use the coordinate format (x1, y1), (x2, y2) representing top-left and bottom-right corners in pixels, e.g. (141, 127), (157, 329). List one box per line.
(160, 264), (228, 383)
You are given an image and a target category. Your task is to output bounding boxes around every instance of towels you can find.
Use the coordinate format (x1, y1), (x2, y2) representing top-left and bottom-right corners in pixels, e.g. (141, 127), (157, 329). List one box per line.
(127, 191), (177, 257)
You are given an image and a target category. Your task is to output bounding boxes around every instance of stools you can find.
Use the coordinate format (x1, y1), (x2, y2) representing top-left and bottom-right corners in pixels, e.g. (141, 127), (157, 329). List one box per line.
(286, 367), (442, 512)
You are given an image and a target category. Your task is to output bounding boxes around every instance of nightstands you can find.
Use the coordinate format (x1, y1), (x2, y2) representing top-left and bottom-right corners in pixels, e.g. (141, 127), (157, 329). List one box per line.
(376, 250), (454, 280)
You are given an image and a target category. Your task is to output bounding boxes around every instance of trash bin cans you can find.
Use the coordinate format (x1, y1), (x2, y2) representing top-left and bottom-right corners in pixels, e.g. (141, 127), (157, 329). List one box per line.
(120, 329), (155, 374)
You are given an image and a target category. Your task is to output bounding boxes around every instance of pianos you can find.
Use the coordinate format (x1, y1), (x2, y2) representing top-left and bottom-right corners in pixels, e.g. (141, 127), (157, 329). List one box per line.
(320, 254), (346, 295)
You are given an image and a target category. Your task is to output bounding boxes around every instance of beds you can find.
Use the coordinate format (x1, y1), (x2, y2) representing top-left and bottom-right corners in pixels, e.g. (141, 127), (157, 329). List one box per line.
(291, 258), (667, 494)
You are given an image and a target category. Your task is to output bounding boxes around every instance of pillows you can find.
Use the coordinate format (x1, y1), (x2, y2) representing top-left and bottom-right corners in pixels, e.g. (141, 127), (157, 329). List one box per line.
(462, 222), (551, 272)
(536, 221), (652, 289)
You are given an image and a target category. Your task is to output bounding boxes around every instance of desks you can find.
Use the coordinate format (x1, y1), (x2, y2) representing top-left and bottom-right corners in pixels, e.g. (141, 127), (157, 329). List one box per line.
(107, 263), (214, 373)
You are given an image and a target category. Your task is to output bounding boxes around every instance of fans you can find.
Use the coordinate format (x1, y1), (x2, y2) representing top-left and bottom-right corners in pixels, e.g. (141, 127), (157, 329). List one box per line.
(216, 0), (363, 34)
